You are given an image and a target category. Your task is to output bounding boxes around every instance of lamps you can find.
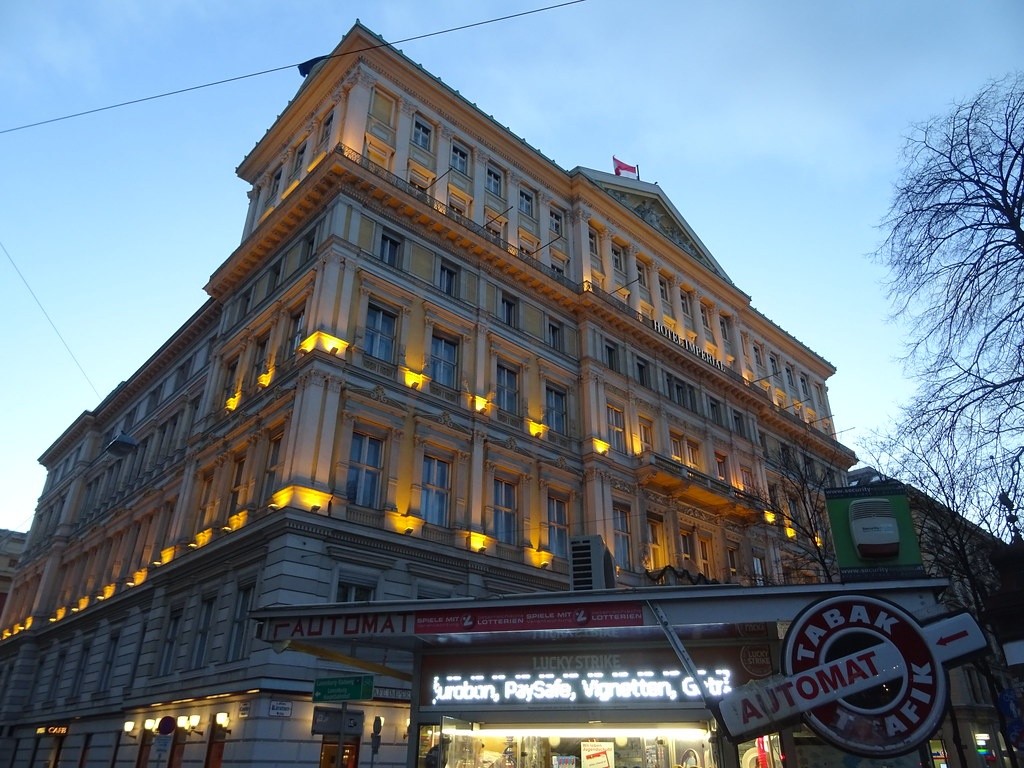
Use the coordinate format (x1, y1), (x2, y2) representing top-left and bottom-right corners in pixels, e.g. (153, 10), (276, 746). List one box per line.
(602, 450), (607, 456)
(268, 503), (280, 512)
(534, 432), (542, 439)
(215, 711), (232, 735)
(151, 561), (162, 568)
(310, 505), (320, 514)
(329, 347), (338, 357)
(477, 546), (487, 554)
(404, 527), (414, 535)
(410, 381), (419, 390)
(540, 562), (549, 570)
(479, 407), (487, 415)
(145, 715), (162, 736)
(177, 715), (204, 737)
(123, 720), (138, 741)
(222, 526), (232, 535)
(187, 544), (197, 550)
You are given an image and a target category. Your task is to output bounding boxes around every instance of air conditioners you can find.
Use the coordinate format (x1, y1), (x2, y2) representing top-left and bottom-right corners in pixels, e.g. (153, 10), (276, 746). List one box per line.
(568, 534), (618, 596)
